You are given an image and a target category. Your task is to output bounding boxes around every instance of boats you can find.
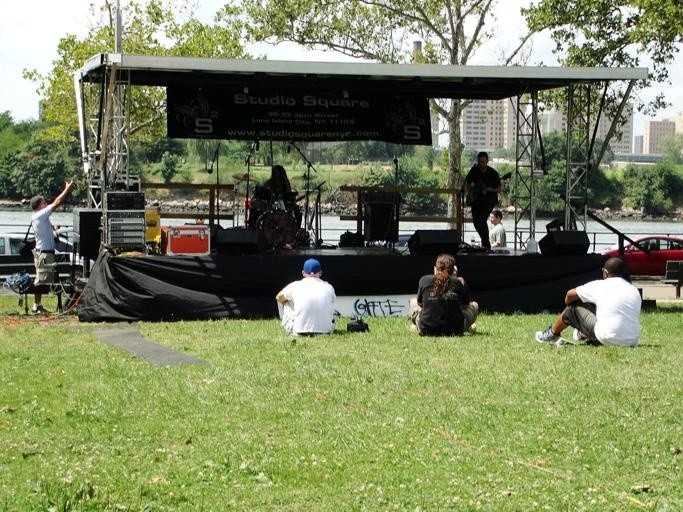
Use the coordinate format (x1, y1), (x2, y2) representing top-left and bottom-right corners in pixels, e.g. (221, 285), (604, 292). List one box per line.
(1, 231), (94, 271)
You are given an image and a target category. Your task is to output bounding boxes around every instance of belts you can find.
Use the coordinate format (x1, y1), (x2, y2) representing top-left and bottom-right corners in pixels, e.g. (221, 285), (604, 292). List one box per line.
(297, 332), (330, 336)
(36, 248), (55, 254)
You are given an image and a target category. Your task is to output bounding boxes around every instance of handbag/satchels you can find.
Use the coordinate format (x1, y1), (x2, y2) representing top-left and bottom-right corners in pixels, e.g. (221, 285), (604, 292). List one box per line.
(3, 273), (35, 294)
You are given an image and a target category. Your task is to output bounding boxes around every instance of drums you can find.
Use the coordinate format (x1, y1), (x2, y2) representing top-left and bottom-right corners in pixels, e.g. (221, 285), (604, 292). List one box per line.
(251, 187), (272, 210)
(256, 208), (297, 246)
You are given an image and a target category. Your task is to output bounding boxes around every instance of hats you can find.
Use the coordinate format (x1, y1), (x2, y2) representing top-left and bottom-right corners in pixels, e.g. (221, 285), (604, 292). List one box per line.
(302, 258), (321, 276)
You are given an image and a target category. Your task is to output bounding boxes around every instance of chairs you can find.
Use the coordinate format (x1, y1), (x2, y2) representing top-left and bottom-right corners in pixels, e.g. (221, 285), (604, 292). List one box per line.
(660, 259), (683, 299)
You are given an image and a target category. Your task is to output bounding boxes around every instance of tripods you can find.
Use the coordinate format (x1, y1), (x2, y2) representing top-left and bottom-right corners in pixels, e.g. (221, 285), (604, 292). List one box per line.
(308, 187), (338, 249)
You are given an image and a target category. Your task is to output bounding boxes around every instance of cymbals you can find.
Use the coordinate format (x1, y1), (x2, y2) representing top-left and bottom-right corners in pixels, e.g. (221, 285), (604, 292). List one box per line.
(291, 175), (316, 180)
(233, 173), (258, 181)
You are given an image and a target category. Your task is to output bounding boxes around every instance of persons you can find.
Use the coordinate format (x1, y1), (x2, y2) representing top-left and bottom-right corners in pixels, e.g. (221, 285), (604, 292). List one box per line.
(262, 165), (292, 250)
(274, 257), (341, 339)
(489, 210), (507, 247)
(534, 256), (643, 347)
(30, 174), (78, 315)
(409, 253), (480, 336)
(465, 152), (502, 250)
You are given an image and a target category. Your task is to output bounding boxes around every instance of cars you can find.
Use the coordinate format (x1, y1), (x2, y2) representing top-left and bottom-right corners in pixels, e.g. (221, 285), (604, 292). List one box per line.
(605, 234), (682, 275)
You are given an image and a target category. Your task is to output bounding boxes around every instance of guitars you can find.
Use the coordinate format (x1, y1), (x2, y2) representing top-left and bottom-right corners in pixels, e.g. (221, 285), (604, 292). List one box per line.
(464, 172), (512, 206)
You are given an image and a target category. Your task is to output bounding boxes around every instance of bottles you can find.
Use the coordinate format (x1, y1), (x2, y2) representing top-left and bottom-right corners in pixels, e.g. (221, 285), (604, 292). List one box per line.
(350, 312), (362, 324)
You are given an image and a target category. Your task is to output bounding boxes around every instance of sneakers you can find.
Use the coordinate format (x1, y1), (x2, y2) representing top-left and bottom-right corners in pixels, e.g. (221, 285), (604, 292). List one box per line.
(411, 324), (420, 333)
(535, 324), (561, 343)
(32, 305), (52, 315)
(463, 322), (476, 336)
(573, 328), (584, 340)
